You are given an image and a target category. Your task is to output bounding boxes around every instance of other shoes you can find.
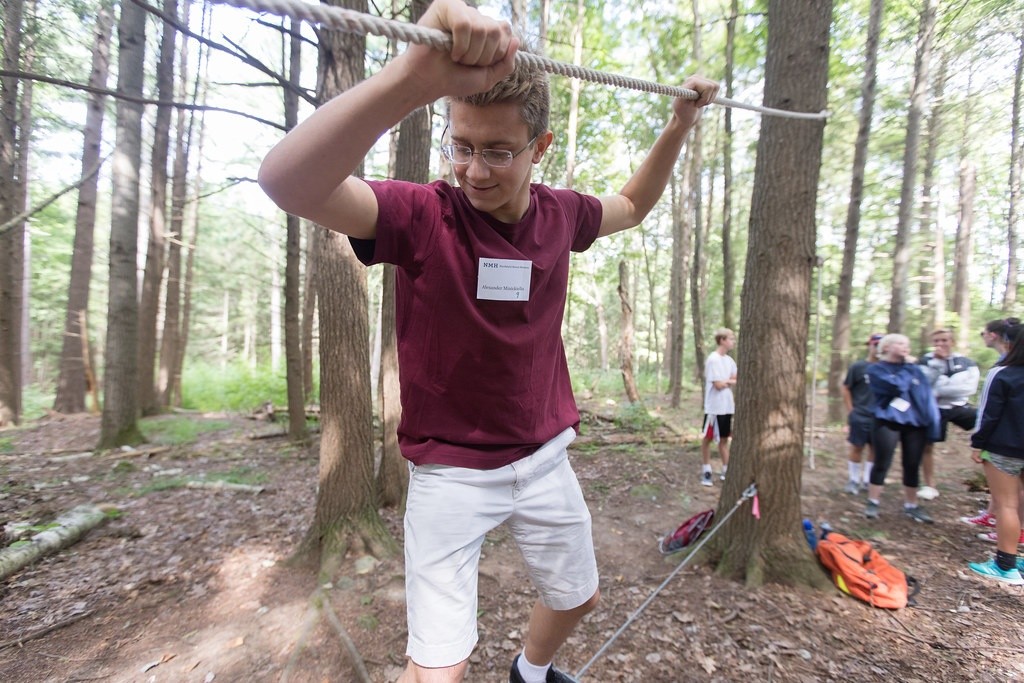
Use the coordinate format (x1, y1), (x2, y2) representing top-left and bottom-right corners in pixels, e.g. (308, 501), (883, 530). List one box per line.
(701, 470), (714, 486)
(906, 504), (933, 524)
(861, 482), (870, 491)
(865, 498), (879, 518)
(508, 653), (577, 683)
(916, 486), (939, 501)
(846, 481), (860, 494)
(1017, 529), (1024, 545)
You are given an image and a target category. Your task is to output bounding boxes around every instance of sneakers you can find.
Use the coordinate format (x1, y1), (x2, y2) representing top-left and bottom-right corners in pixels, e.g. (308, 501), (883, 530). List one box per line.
(977, 533), (997, 541)
(961, 512), (997, 532)
(969, 558), (1024, 584)
(1015, 557), (1024, 571)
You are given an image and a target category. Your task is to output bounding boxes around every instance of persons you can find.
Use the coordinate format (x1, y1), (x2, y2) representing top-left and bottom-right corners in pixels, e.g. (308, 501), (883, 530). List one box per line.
(700, 328), (736, 486)
(258, 0), (721, 683)
(839, 313), (1024, 585)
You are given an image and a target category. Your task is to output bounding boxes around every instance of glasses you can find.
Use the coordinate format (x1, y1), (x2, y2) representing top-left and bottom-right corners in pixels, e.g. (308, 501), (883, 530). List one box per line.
(875, 342), (879, 346)
(440, 124), (542, 168)
(981, 332), (983, 335)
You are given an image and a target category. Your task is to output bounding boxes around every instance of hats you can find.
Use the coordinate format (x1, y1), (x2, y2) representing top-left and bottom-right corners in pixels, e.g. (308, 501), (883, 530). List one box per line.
(865, 334), (884, 345)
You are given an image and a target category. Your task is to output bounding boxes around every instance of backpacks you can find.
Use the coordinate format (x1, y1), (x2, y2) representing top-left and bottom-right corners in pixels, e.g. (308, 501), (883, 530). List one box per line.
(817, 531), (920, 609)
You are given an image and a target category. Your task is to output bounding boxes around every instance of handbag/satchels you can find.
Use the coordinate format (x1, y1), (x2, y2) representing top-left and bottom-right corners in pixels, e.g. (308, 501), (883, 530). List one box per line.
(659, 509), (715, 554)
